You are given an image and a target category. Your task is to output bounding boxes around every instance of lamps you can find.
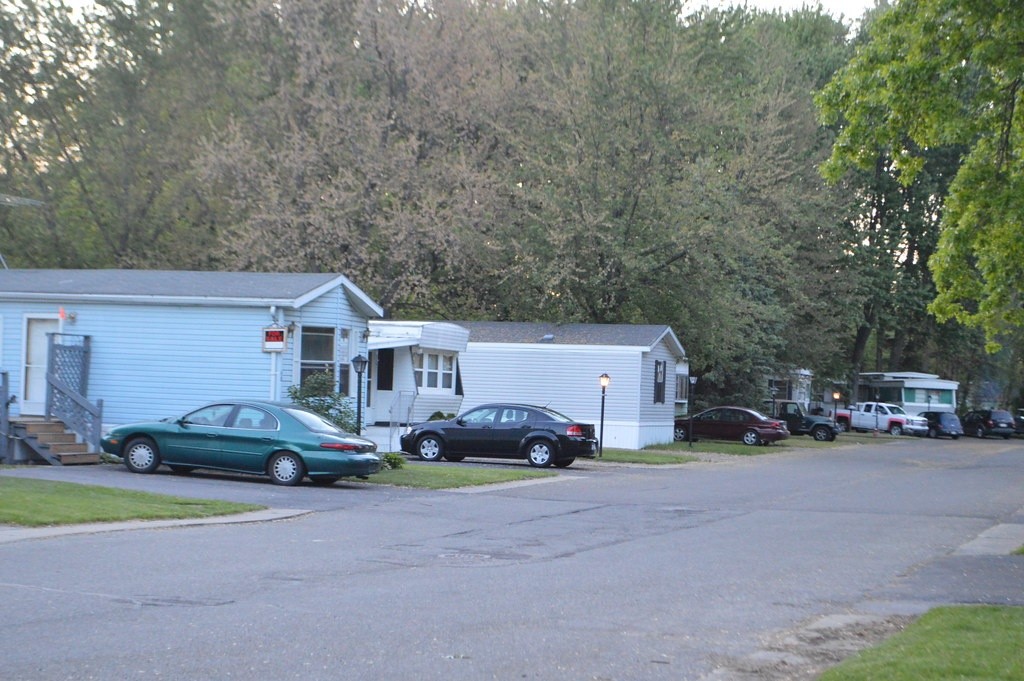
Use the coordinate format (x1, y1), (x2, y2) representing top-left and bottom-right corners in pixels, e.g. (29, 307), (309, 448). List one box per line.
(360, 327), (370, 344)
(288, 321), (298, 337)
(412, 349), (423, 358)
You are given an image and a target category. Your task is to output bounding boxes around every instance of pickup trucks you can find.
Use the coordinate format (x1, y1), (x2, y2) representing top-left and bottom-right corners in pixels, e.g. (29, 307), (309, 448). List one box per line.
(830, 402), (930, 438)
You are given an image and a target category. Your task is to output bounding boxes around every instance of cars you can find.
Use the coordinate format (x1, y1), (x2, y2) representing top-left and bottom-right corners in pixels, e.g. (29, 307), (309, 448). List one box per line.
(99, 399), (382, 486)
(962, 408), (1016, 439)
(914, 411), (965, 439)
(674, 406), (791, 446)
(400, 402), (598, 469)
(1013, 416), (1024, 434)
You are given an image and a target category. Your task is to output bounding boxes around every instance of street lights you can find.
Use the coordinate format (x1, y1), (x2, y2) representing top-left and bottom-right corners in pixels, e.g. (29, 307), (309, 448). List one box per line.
(926, 395), (932, 412)
(689, 371), (698, 448)
(351, 354), (369, 436)
(772, 387), (776, 419)
(832, 390), (840, 429)
(598, 373), (612, 457)
(874, 392), (880, 432)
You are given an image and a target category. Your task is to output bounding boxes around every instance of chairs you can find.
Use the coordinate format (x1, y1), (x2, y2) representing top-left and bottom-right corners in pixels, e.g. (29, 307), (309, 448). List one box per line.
(239, 419), (252, 428)
(505, 409), (525, 422)
(258, 418), (274, 428)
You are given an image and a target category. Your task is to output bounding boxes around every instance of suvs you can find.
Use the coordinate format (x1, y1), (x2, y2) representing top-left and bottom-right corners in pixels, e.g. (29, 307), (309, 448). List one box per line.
(758, 399), (841, 442)
